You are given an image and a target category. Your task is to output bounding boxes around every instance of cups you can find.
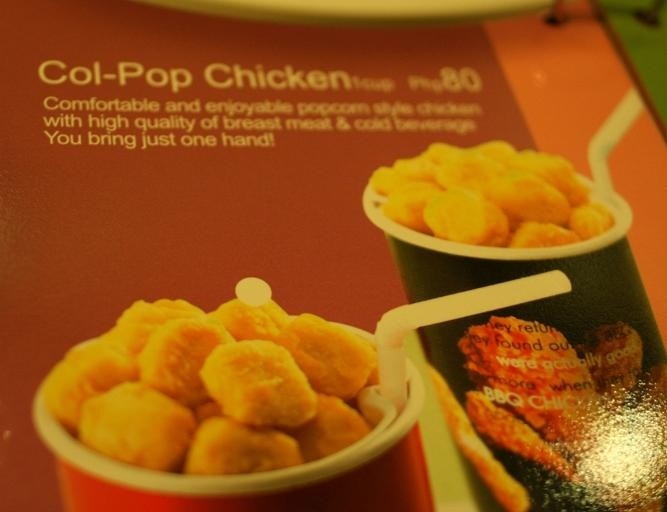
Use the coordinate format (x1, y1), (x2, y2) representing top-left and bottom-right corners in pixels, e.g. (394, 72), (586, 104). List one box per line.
(363, 174), (667, 509)
(31, 316), (425, 512)
